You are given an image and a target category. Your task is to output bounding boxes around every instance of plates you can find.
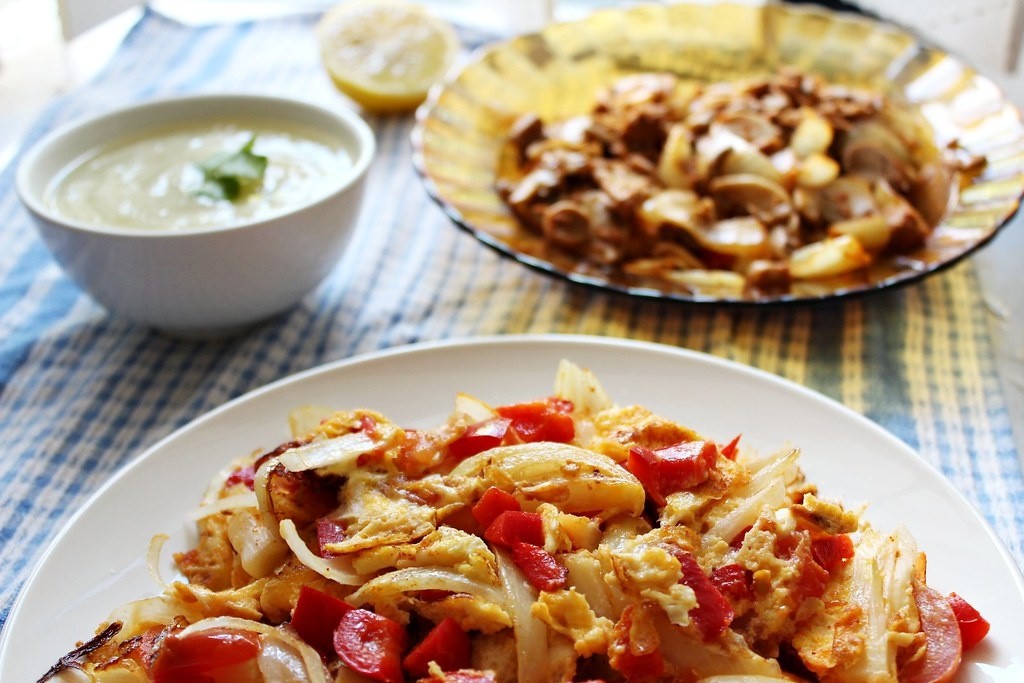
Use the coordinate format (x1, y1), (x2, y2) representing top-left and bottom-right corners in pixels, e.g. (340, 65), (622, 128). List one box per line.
(410, 0), (1023, 307)
(5, 336), (1024, 682)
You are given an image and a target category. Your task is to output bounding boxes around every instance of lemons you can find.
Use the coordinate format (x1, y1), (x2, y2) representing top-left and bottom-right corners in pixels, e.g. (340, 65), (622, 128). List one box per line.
(319, 0), (452, 116)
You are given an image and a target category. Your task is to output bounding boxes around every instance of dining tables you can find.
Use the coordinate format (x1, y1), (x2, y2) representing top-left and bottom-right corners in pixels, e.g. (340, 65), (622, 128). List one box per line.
(0, 0), (1024, 683)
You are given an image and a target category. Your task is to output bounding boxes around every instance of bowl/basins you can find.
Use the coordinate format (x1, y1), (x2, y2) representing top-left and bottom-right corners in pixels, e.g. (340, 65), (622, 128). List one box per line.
(14, 89), (377, 339)
